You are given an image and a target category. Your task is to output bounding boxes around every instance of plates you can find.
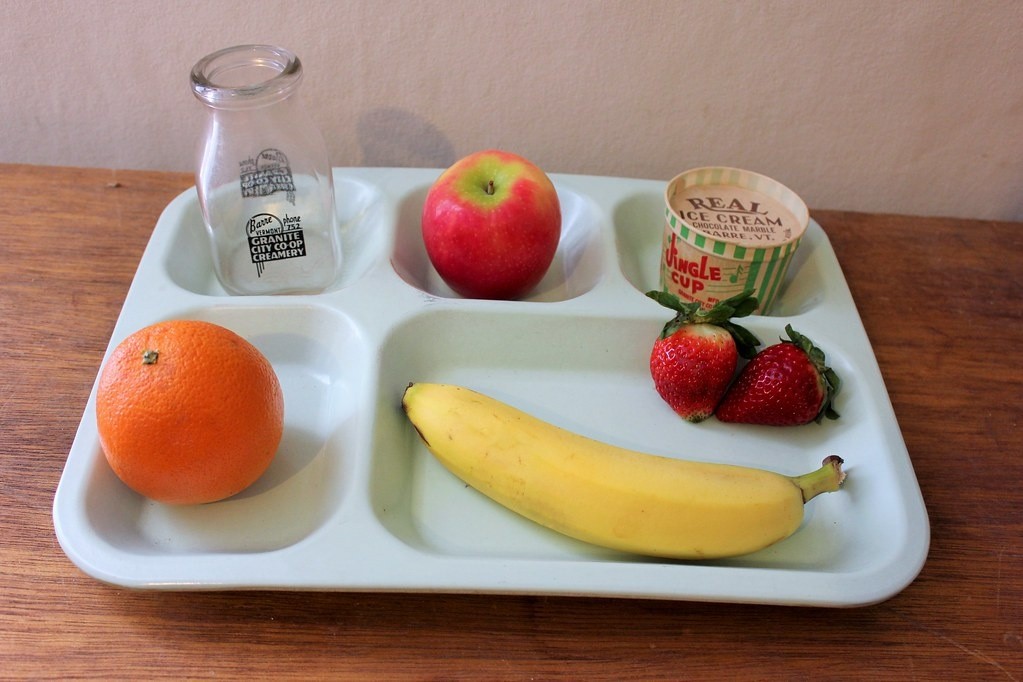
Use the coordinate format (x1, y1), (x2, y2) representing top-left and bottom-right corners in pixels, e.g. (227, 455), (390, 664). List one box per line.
(52, 155), (937, 611)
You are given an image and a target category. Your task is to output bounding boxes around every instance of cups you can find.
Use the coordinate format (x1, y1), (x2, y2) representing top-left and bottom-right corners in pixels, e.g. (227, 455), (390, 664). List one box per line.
(657, 169), (811, 314)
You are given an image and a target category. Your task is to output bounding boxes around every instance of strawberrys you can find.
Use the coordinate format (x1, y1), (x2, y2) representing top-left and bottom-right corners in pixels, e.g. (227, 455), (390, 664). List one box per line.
(644, 289), (844, 428)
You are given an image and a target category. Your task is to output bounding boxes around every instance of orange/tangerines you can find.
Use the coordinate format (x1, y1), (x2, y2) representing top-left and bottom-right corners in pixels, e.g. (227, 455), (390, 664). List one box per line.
(95, 321), (284, 505)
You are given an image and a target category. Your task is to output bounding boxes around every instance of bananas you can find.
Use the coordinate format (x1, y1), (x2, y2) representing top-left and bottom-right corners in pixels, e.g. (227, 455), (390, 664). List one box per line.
(401, 381), (847, 559)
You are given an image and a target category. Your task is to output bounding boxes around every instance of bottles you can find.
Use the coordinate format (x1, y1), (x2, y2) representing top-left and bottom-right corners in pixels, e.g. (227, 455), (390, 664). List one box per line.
(190, 44), (344, 295)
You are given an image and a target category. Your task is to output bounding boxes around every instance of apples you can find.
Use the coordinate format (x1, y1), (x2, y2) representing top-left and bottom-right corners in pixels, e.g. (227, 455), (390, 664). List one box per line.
(421, 149), (561, 301)
